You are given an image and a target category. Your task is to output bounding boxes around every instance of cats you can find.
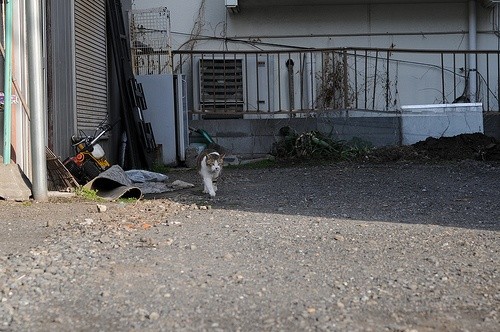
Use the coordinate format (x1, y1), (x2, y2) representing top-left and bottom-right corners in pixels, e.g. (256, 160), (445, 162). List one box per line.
(197, 149), (226, 196)
